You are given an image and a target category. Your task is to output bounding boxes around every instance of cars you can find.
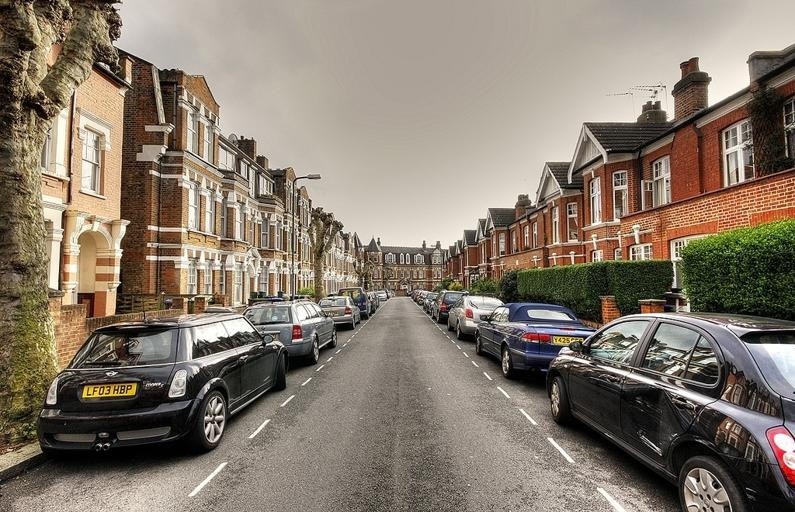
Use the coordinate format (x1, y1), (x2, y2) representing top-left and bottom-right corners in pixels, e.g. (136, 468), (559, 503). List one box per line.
(317, 286), (395, 329)
(407, 288), (506, 340)
(37, 282), (290, 451)
(547, 312), (795, 512)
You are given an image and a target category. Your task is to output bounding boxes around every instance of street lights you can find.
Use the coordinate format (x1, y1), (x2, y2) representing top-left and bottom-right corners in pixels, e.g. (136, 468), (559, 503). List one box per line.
(290, 173), (322, 298)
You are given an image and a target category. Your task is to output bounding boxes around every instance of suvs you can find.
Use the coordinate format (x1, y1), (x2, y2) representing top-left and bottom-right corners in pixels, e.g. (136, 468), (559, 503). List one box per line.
(242, 299), (337, 364)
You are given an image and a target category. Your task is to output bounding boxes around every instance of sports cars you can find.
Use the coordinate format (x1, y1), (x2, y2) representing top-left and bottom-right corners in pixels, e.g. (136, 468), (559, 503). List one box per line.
(475, 301), (598, 378)
